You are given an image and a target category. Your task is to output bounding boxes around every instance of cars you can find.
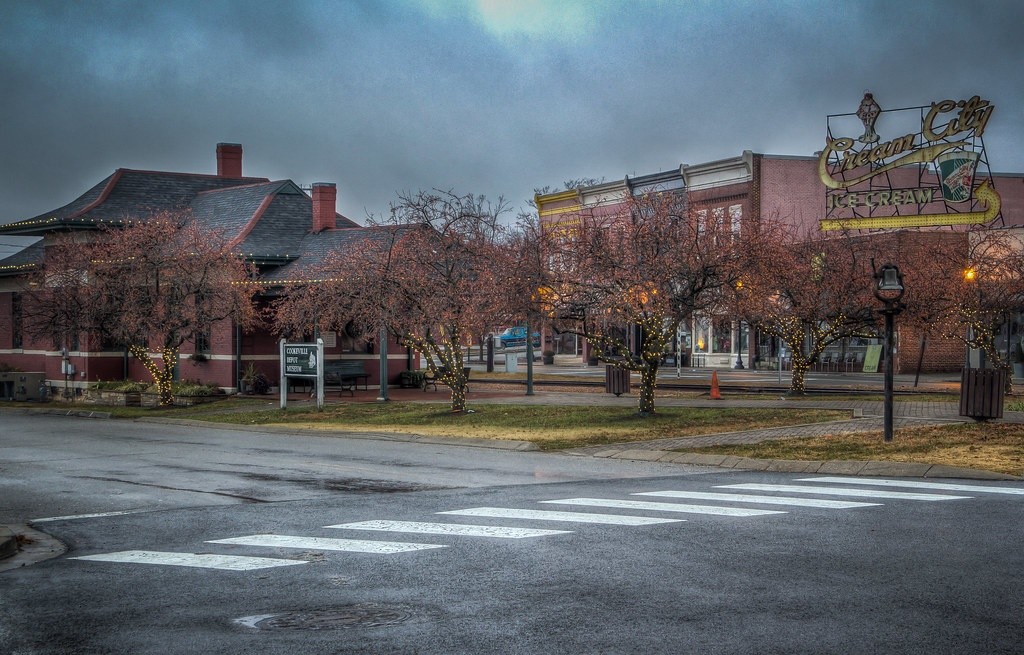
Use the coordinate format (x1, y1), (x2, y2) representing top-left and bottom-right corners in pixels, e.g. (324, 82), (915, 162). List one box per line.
(484, 327), (542, 351)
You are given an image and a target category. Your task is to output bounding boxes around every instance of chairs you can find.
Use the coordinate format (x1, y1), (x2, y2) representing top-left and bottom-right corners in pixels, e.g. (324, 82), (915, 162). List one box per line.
(785, 352), (864, 373)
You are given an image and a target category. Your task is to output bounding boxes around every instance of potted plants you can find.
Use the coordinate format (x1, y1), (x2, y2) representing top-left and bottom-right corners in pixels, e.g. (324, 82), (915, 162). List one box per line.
(399, 369), (423, 387)
(240, 362), (272, 394)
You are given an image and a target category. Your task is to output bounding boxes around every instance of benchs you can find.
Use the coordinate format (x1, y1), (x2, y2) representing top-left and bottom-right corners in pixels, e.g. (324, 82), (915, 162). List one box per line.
(285, 363), (372, 396)
(423, 367), (471, 394)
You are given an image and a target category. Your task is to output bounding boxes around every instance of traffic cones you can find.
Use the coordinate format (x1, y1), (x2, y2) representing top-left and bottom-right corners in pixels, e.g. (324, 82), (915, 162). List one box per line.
(708, 371), (726, 400)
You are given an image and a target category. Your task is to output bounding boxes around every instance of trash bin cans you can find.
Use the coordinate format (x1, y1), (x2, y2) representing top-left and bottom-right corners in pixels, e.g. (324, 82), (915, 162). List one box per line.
(959, 367), (1006, 418)
(606, 365), (630, 393)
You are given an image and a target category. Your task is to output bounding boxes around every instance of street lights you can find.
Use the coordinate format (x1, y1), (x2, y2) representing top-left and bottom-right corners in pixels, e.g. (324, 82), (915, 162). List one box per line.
(874, 262), (905, 441)
(966, 267), (976, 369)
(735, 278), (745, 369)
